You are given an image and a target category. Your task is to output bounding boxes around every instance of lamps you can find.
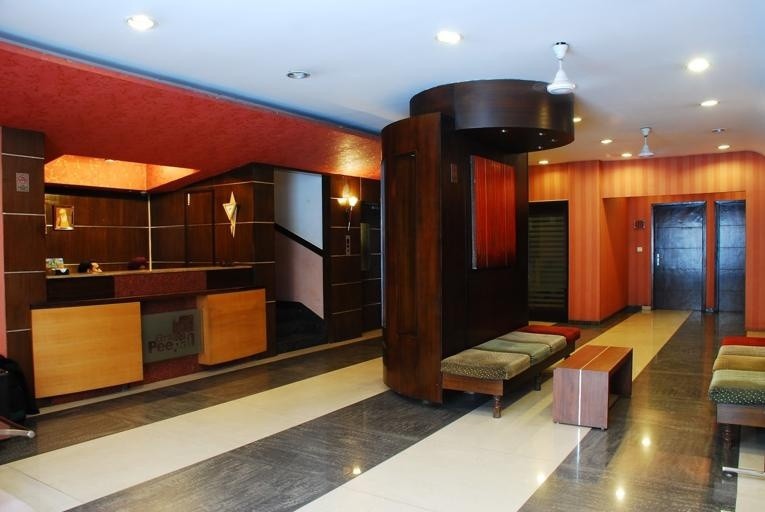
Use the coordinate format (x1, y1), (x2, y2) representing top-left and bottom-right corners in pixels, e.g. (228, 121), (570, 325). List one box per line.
(547, 42), (576, 95)
(638, 127), (655, 158)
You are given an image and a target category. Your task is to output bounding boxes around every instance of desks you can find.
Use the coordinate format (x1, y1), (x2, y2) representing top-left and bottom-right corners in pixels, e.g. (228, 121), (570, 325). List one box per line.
(552, 345), (633, 431)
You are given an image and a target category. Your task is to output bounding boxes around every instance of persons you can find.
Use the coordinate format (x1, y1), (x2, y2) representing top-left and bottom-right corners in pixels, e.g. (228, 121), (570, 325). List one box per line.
(77, 259), (104, 275)
(128, 256), (147, 271)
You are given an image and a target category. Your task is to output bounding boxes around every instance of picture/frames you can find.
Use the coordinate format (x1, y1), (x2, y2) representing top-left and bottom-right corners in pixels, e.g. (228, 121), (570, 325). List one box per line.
(52, 204), (75, 231)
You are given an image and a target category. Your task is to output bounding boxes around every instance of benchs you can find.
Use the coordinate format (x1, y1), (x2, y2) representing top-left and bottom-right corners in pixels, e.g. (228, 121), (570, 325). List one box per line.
(441, 324), (581, 418)
(707, 337), (765, 450)
